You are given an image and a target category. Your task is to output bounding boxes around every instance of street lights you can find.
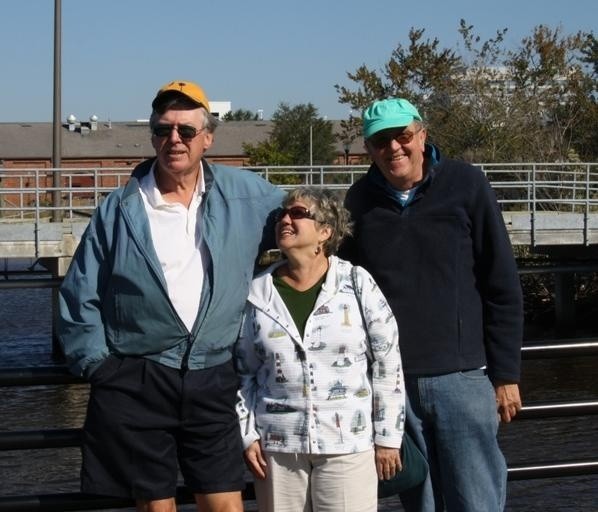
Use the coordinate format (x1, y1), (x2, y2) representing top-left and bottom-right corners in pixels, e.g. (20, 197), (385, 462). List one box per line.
(308, 114), (317, 184)
(341, 142), (352, 165)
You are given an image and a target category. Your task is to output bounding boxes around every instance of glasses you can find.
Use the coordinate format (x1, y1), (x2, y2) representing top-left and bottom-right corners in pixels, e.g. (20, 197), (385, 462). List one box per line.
(272, 202), (331, 224)
(366, 125), (425, 150)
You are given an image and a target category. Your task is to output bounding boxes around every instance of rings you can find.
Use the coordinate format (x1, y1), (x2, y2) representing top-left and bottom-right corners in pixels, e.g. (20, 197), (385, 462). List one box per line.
(389, 464), (397, 469)
(510, 407), (515, 410)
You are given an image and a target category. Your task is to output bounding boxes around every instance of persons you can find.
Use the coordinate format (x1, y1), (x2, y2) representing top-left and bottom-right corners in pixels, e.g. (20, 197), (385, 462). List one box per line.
(55, 81), (292, 511)
(235, 186), (406, 511)
(336, 99), (525, 511)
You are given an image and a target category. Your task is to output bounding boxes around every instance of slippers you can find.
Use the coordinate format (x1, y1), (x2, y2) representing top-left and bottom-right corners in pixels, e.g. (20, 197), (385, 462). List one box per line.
(152, 121), (212, 140)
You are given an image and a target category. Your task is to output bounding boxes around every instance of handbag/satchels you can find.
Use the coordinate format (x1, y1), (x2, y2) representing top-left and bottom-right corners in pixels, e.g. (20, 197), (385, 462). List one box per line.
(363, 355), (433, 501)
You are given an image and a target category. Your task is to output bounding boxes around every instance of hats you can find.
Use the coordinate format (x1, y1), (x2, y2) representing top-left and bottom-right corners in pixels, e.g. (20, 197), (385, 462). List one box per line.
(152, 77), (213, 120)
(360, 96), (427, 142)
